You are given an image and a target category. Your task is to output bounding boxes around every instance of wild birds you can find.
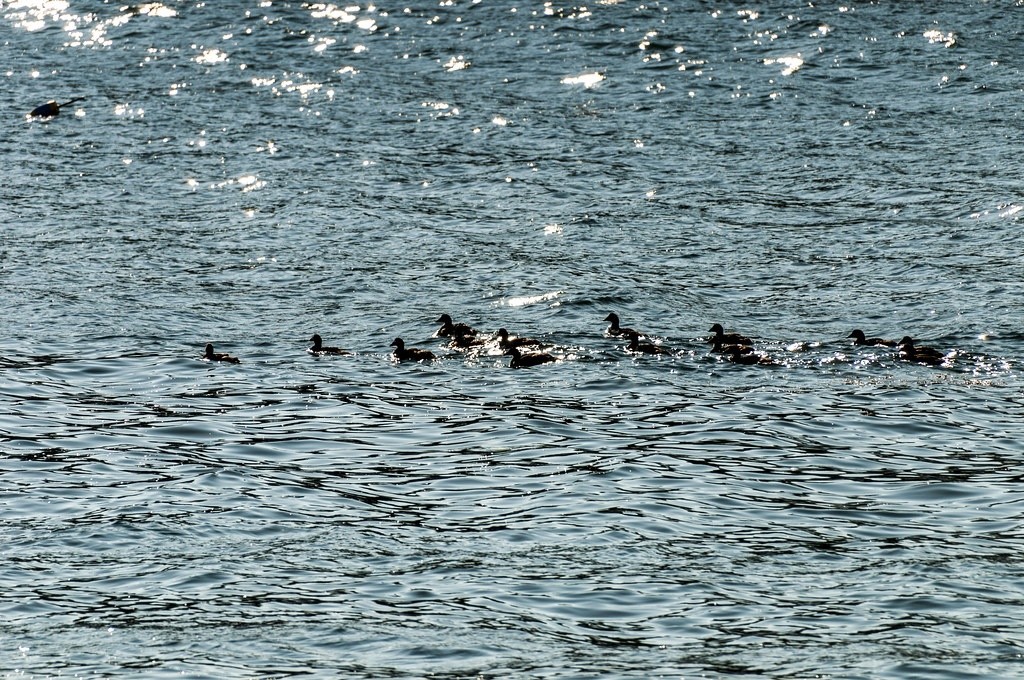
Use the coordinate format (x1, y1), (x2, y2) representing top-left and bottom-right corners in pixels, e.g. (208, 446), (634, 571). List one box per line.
(308, 334), (345, 355)
(202, 343), (240, 364)
(433, 313), (557, 370)
(706, 323), (762, 366)
(602, 313), (671, 356)
(390, 337), (436, 360)
(846, 329), (946, 366)
